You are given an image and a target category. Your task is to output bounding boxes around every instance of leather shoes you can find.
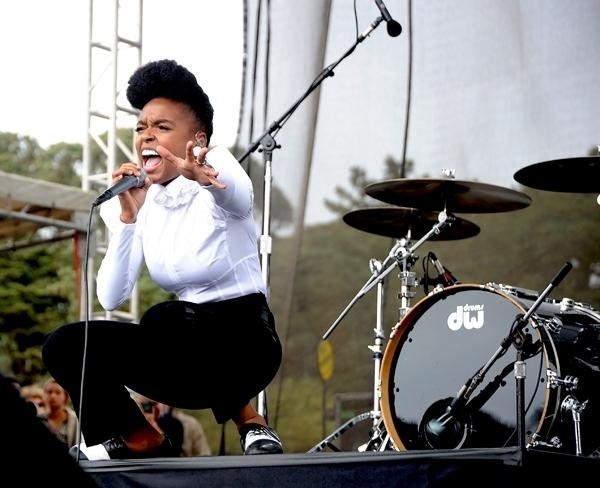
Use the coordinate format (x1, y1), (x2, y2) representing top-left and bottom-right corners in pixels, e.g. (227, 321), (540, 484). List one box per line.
(240, 423), (285, 454)
(66, 426), (175, 463)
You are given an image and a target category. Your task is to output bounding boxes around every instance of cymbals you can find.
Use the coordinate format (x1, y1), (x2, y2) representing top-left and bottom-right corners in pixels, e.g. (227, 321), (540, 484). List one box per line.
(514, 157), (599, 194)
(342, 205), (480, 241)
(363, 178), (532, 214)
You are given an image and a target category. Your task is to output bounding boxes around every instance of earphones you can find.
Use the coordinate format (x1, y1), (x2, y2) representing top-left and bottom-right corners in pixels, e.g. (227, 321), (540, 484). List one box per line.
(197, 138), (204, 147)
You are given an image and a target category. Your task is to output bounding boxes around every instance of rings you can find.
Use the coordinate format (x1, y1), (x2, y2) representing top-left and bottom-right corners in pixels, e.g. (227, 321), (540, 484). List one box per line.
(195, 159), (207, 165)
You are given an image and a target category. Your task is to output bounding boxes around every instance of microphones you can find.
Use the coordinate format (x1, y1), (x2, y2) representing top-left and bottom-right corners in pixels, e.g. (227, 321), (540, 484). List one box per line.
(93, 167), (148, 207)
(429, 253), (461, 286)
(446, 376), (472, 413)
(374, 0), (402, 37)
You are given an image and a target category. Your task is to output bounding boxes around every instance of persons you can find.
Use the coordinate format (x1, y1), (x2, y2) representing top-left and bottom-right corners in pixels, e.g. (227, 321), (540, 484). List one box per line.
(162, 399), (213, 456)
(125, 386), (184, 458)
(38, 56), (290, 460)
(43, 378), (81, 449)
(16, 380), (51, 428)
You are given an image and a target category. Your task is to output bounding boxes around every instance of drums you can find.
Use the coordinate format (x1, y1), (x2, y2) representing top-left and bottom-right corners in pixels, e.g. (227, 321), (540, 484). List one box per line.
(378, 283), (600, 451)
